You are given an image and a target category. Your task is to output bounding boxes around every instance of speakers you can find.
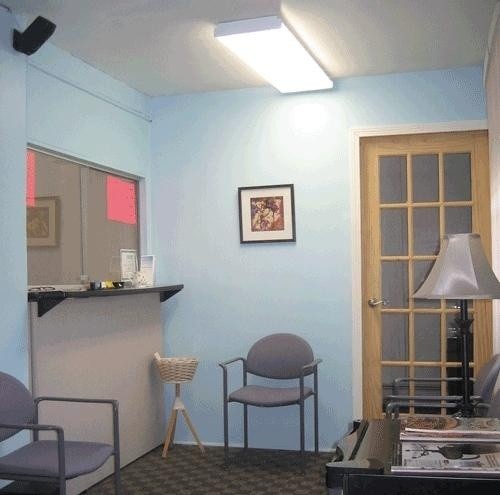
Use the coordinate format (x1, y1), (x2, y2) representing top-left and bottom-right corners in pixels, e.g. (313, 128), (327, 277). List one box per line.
(15, 16), (56, 55)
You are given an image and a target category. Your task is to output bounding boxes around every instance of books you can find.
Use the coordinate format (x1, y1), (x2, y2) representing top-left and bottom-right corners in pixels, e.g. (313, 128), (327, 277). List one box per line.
(391, 414), (500, 475)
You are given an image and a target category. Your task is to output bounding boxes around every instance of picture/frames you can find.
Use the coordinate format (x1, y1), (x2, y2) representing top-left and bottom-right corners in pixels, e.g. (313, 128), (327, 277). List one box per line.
(27, 196), (62, 247)
(237, 183), (297, 245)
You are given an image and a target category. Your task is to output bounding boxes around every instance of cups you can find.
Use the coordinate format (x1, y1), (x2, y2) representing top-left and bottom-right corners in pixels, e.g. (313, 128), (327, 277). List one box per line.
(80, 274), (124, 290)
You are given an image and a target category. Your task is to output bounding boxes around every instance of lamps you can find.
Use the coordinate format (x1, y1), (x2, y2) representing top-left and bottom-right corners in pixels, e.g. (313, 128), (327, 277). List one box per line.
(417, 233), (499, 417)
(212, 17), (335, 94)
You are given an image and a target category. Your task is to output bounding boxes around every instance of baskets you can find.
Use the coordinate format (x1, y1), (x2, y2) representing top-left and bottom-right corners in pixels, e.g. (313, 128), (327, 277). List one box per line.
(156, 356), (201, 384)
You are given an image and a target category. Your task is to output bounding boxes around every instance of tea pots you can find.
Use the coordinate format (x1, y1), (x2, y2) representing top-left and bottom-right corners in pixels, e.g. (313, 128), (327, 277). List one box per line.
(128, 271), (150, 288)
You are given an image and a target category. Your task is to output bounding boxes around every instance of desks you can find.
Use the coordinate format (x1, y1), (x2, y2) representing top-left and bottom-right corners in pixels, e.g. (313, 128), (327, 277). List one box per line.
(327, 416), (500, 493)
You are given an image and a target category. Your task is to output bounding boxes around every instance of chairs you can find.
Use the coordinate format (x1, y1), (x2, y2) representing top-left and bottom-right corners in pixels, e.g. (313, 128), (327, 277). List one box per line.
(2, 368), (124, 494)
(219, 332), (323, 480)
(386, 387), (500, 418)
(384, 351), (500, 417)
